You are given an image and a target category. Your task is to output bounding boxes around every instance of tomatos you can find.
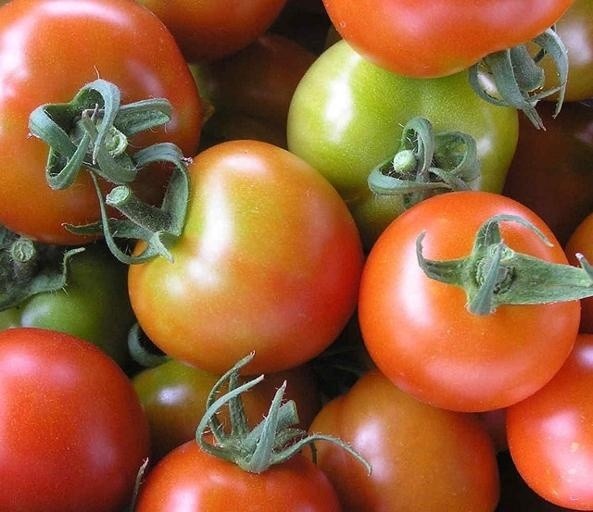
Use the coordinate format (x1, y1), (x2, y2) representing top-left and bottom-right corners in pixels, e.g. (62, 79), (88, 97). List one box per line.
(1, 0), (593, 511)
